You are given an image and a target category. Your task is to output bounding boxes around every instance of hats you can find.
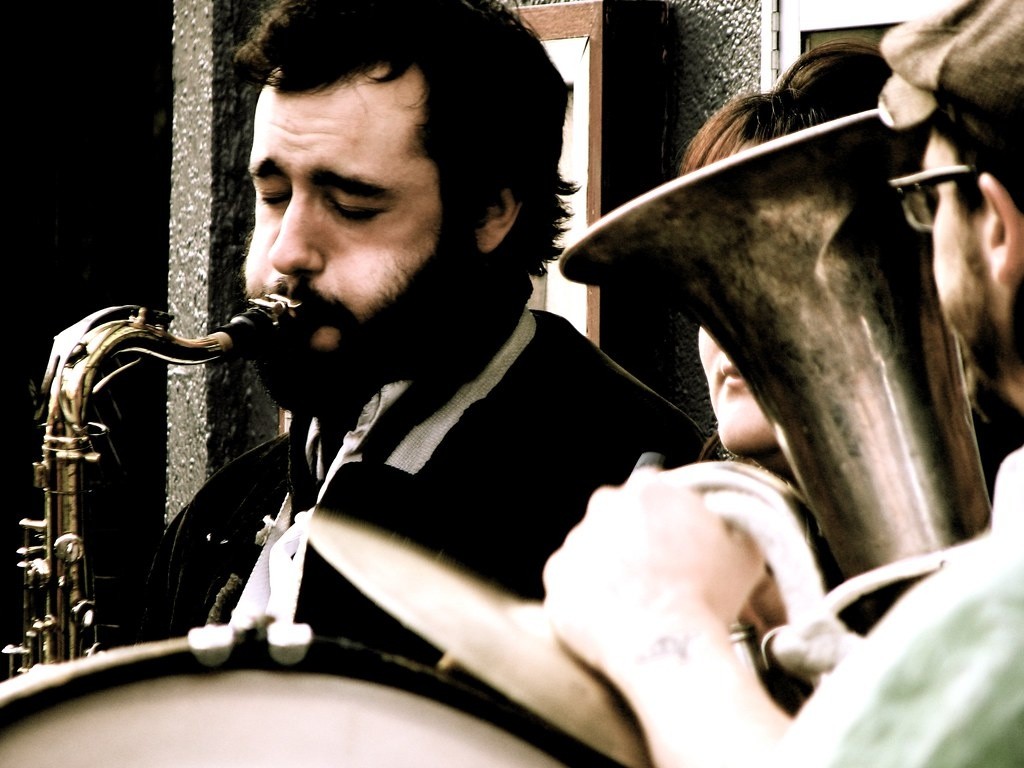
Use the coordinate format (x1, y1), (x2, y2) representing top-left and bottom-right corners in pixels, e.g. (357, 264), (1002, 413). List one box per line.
(876, 0), (1024, 166)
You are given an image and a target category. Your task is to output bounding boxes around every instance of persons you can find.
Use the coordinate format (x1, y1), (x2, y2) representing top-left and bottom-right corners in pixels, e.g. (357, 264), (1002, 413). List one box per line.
(104, 0), (734, 672)
(544, 0), (1024, 768)
(675, 40), (1023, 716)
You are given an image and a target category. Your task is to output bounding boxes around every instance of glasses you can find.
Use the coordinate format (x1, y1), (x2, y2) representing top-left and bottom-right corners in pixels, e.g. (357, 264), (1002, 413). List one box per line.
(890, 167), (1005, 231)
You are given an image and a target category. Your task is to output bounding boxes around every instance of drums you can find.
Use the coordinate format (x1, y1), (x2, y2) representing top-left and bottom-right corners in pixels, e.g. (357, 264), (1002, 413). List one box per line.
(1, 619), (624, 766)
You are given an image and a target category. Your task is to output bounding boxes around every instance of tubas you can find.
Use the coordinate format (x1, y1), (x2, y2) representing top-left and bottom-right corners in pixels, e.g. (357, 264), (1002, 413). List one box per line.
(554, 105), (994, 622)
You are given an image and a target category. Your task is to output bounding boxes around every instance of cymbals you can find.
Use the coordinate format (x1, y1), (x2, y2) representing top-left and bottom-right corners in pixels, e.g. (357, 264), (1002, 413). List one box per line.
(299, 512), (657, 767)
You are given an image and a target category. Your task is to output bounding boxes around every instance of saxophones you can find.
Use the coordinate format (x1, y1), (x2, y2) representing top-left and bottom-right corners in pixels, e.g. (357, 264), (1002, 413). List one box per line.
(3, 294), (306, 674)
